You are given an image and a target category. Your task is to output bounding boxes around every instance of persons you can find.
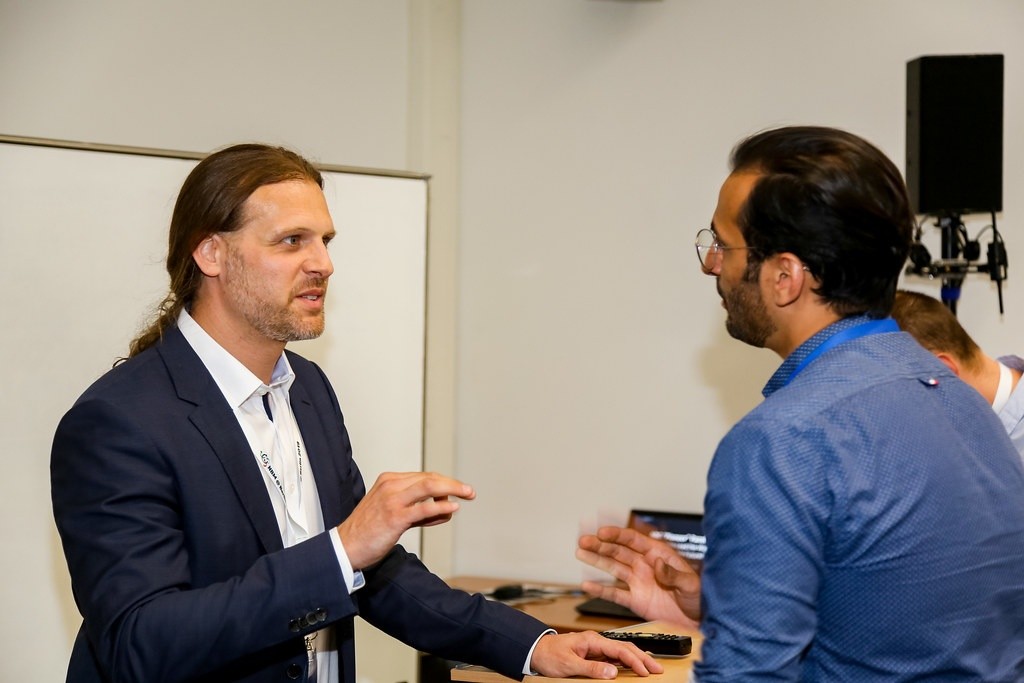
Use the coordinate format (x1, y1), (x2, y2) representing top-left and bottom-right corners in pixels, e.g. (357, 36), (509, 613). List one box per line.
(51, 145), (663, 683)
(576, 125), (1024, 683)
(896, 289), (1024, 462)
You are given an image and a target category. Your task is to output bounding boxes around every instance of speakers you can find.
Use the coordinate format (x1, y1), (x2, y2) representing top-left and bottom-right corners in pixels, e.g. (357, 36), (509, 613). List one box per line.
(902, 53), (1003, 217)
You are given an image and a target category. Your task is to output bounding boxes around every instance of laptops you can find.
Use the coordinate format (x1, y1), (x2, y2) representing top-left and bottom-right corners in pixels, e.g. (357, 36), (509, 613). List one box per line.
(575, 509), (708, 622)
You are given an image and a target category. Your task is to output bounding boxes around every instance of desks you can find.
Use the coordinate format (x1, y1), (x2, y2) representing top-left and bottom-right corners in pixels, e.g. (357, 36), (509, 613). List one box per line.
(416, 577), (706, 683)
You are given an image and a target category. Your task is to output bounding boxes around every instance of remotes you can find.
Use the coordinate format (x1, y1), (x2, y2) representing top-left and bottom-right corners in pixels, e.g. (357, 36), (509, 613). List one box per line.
(599, 631), (693, 658)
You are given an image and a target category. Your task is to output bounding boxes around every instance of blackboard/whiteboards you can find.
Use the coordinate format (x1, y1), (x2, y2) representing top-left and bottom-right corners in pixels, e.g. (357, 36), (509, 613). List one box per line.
(2, 133), (430, 683)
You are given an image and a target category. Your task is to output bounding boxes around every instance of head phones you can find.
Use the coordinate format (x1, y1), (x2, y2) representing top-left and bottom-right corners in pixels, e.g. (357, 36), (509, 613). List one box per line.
(911, 216), (980, 269)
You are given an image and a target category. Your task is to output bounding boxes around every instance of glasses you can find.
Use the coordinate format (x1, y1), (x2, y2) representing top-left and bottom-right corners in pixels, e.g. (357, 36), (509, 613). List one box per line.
(694, 228), (757, 272)
